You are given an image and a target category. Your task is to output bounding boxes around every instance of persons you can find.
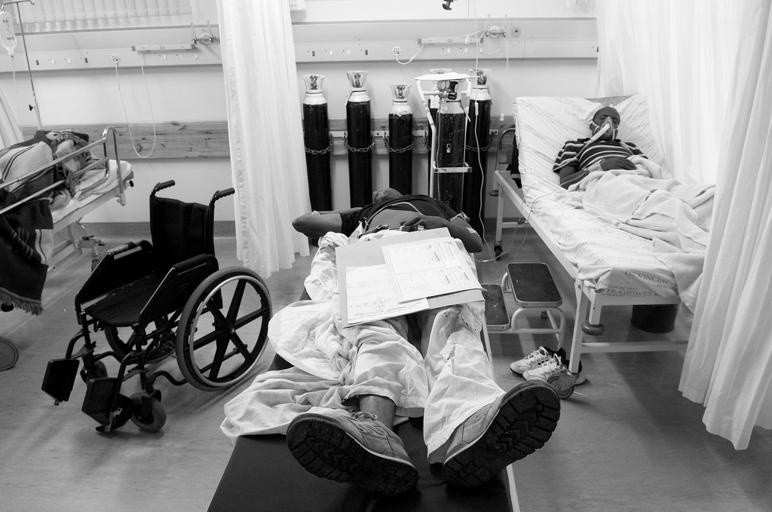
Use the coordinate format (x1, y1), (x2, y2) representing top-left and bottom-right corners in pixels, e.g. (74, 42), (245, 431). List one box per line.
(285, 188), (561, 496)
(553, 106), (649, 189)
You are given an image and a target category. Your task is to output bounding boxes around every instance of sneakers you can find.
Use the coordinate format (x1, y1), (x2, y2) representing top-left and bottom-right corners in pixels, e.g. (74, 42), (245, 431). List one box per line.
(440, 381), (562, 492)
(522, 353), (583, 393)
(510, 346), (567, 376)
(284, 412), (420, 495)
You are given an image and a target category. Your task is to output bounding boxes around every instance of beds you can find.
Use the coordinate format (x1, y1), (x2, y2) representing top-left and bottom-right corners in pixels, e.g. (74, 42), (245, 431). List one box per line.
(202, 233), (518, 511)
(493, 129), (713, 383)
(0, 153), (134, 370)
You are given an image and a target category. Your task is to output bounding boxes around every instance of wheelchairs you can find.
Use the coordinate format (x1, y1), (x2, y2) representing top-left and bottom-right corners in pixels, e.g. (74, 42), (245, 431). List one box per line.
(43, 181), (273, 433)
